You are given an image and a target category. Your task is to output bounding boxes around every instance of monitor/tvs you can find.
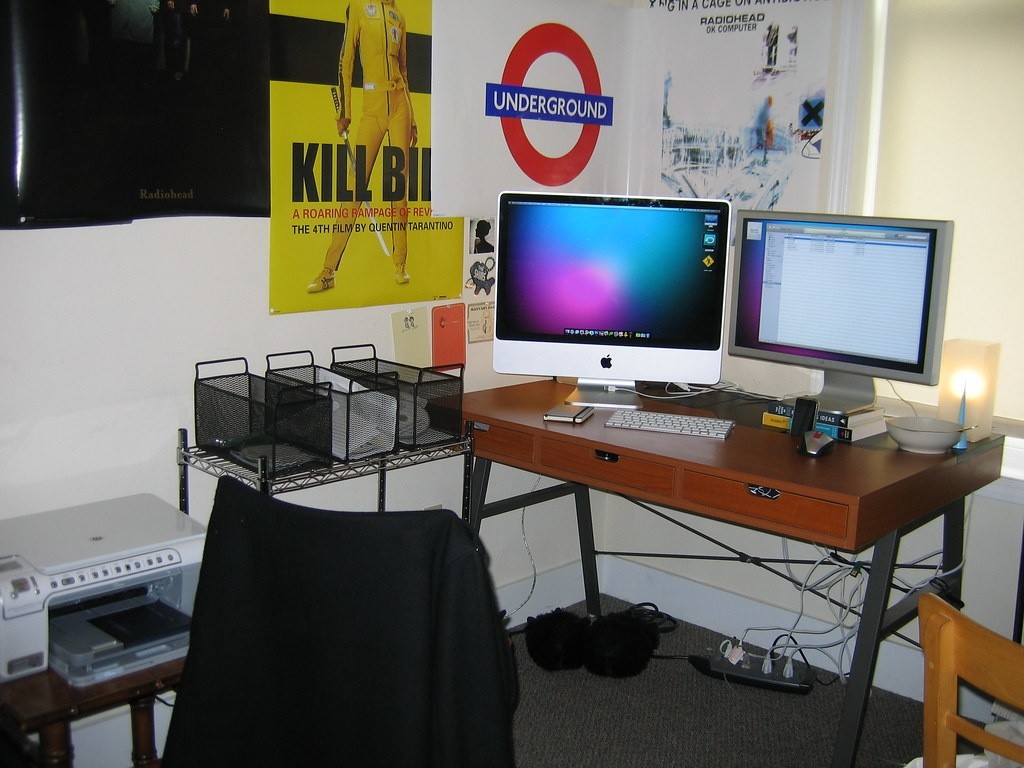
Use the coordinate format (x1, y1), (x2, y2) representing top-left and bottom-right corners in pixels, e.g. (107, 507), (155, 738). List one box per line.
(727, 209), (955, 416)
(493, 193), (730, 408)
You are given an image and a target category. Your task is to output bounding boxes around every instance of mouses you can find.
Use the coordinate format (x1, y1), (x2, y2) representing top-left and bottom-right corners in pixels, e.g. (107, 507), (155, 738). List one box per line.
(795, 430), (835, 457)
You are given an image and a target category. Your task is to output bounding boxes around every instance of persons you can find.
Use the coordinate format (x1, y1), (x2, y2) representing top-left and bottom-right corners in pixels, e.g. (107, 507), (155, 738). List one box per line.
(306, 0), (419, 293)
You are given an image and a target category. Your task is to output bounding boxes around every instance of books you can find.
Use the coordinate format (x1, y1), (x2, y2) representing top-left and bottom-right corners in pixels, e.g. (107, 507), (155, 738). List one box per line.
(761, 411), (888, 442)
(768, 399), (885, 428)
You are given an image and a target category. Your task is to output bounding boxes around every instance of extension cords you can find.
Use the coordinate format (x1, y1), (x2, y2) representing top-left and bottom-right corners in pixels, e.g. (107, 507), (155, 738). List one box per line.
(710, 651), (821, 693)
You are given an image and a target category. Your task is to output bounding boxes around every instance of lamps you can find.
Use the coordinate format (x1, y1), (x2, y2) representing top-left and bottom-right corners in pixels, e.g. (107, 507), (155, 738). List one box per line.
(937, 337), (1001, 442)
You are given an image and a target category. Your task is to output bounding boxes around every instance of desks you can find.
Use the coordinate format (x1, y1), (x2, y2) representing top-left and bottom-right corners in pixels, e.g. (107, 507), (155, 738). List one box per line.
(0, 657), (187, 768)
(426, 379), (1005, 768)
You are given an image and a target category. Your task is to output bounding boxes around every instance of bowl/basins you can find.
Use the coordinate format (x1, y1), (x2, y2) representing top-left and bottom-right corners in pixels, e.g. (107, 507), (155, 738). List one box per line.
(885, 417), (963, 455)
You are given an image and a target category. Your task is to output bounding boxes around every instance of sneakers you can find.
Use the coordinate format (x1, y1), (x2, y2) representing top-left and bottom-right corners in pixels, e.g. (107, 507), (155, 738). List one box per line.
(307, 271), (333, 291)
(394, 262), (409, 284)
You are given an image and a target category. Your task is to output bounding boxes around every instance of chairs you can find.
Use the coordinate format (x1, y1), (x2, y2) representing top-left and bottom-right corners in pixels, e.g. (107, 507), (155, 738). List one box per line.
(917, 591), (1024, 768)
(160, 475), (518, 768)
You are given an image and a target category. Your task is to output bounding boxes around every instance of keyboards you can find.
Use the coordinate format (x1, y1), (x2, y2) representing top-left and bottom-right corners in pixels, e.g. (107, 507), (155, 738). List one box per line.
(604, 408), (736, 439)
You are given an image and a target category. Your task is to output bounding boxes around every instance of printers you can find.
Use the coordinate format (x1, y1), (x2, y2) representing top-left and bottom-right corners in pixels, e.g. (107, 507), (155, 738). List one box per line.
(1, 494), (208, 691)
